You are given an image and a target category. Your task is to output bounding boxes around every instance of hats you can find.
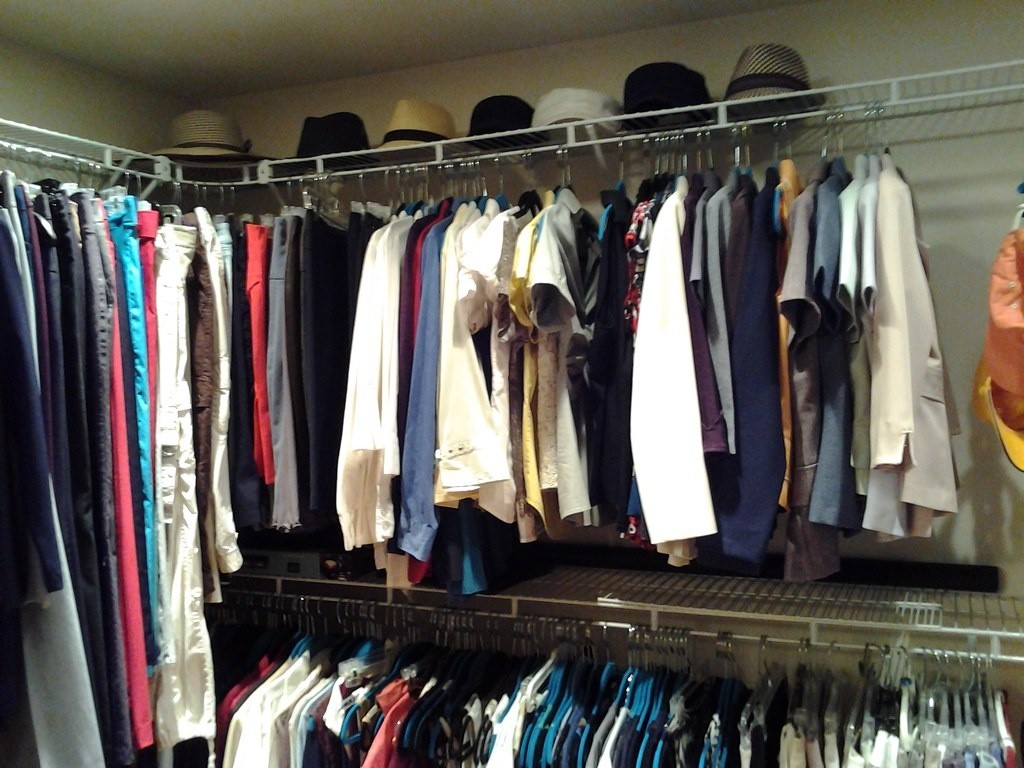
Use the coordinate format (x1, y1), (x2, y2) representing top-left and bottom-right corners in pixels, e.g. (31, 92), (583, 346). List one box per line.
(112, 109), (282, 182)
(723, 44), (827, 113)
(623, 62), (714, 130)
(463, 95), (550, 150)
(374, 100), (456, 150)
(284, 111), (380, 166)
(532, 88), (624, 135)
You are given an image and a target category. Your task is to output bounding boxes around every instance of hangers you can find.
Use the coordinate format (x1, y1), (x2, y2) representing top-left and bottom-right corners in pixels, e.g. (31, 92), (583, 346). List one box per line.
(214, 591), (1016, 762)
(0, 98), (900, 226)
